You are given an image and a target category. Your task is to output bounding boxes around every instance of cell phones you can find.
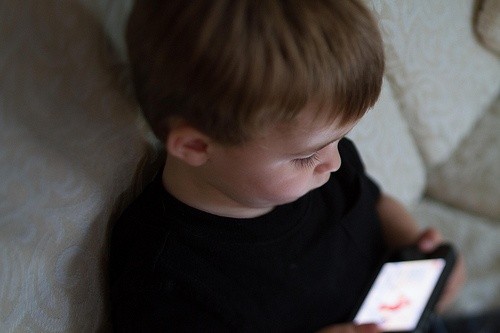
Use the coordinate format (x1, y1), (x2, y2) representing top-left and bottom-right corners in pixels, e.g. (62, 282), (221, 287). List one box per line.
(350, 242), (457, 333)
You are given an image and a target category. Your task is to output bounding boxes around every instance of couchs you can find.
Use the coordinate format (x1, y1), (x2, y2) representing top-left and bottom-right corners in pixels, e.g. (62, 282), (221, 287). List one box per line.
(0, 0), (500, 333)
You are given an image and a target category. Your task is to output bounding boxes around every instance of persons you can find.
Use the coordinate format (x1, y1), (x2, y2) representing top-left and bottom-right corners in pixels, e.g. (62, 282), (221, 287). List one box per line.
(100, 0), (469, 333)
